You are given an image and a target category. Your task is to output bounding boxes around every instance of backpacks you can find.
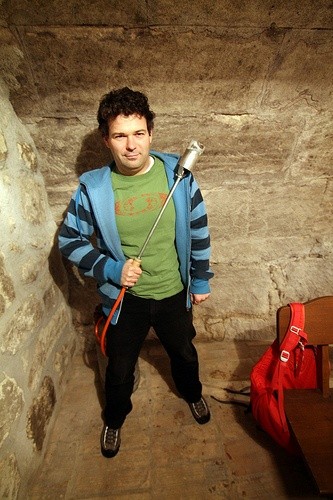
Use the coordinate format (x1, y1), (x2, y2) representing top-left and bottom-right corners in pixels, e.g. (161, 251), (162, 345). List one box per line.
(249, 303), (331, 455)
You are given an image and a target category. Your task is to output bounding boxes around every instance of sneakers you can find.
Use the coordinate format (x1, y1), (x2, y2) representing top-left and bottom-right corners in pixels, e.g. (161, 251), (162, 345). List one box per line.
(101, 423), (121, 458)
(189, 396), (210, 424)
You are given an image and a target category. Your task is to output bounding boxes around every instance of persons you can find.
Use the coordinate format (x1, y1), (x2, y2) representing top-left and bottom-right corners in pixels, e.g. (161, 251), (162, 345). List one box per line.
(53, 87), (212, 459)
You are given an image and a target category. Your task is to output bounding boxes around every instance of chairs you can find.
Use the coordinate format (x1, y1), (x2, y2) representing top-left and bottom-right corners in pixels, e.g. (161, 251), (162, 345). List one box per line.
(272, 295), (333, 500)
